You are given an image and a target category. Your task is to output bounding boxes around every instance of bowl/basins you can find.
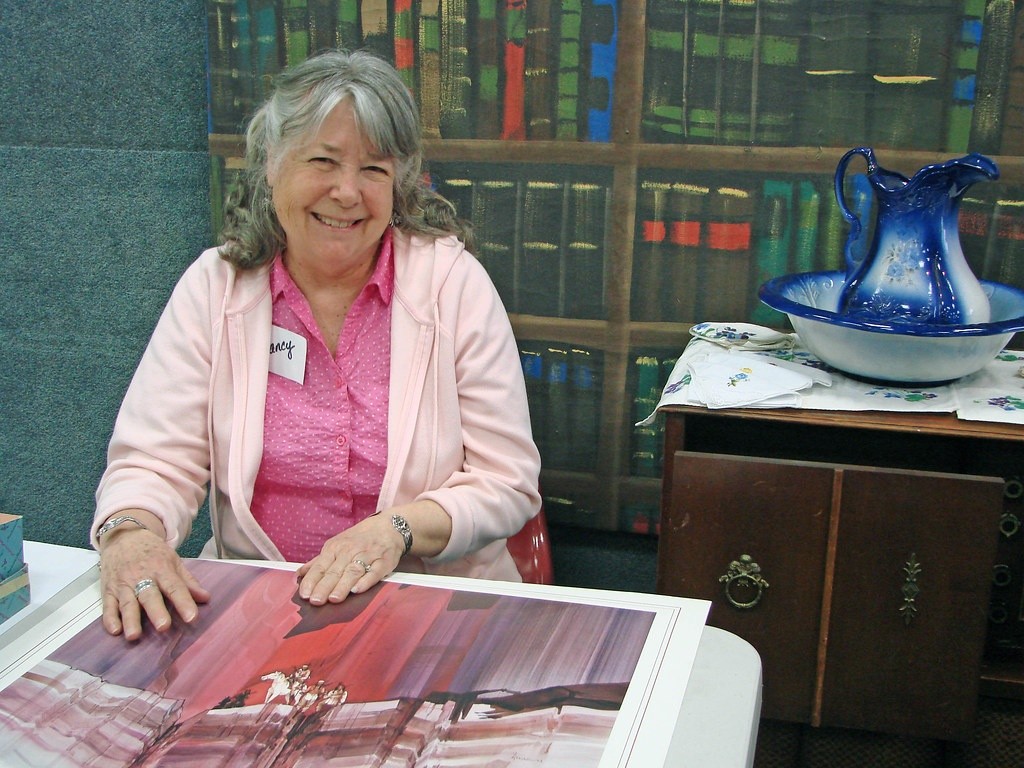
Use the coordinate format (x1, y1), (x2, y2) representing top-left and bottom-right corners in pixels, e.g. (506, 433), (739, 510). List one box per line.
(757, 268), (1023, 382)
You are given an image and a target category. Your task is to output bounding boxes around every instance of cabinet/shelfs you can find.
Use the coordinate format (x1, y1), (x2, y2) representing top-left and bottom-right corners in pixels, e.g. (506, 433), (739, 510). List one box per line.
(656, 324), (1024, 768)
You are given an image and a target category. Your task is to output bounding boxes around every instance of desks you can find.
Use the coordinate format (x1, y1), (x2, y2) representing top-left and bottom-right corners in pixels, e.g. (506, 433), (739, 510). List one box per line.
(0, 540), (762, 768)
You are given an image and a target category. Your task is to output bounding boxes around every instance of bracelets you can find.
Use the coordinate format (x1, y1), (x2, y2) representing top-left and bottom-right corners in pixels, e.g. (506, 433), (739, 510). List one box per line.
(96, 515), (151, 571)
(367, 510), (413, 559)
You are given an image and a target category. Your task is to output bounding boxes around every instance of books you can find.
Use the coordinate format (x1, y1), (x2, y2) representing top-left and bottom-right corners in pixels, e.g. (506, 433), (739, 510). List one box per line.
(202, 0), (1024, 536)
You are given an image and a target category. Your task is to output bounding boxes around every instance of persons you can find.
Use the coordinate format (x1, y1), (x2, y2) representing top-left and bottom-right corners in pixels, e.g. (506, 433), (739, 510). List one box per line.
(91, 51), (541, 642)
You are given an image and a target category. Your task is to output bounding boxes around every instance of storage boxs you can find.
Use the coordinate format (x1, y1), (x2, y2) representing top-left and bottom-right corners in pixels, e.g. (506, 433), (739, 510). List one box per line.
(0, 513), (31, 625)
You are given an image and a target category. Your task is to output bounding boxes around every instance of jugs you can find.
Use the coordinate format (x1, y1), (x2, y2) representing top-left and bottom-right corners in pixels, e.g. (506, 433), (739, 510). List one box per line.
(834, 148), (1000, 325)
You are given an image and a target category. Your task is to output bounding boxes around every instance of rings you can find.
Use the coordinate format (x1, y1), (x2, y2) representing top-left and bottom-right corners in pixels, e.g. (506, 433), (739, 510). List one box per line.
(350, 560), (371, 574)
(133, 577), (159, 599)
